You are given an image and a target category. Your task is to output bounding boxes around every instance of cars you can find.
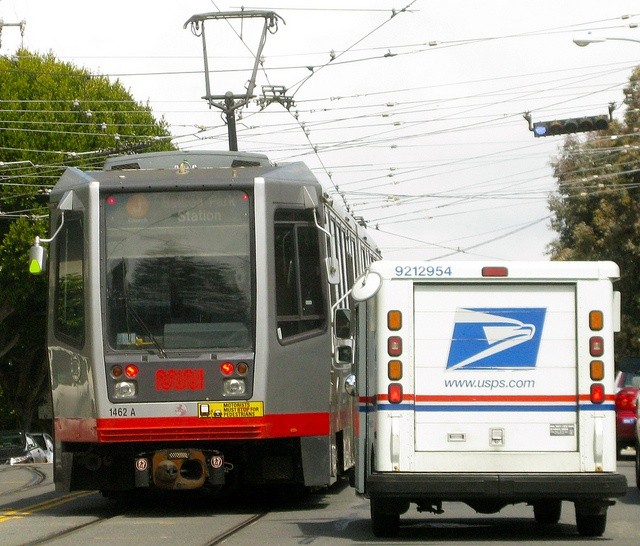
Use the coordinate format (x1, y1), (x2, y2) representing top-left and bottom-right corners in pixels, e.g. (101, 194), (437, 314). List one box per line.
(28, 431), (54, 463)
(0, 432), (48, 465)
(613, 371), (640, 459)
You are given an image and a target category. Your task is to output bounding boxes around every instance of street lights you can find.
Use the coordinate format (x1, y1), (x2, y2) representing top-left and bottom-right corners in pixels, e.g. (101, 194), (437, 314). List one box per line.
(572, 38), (640, 46)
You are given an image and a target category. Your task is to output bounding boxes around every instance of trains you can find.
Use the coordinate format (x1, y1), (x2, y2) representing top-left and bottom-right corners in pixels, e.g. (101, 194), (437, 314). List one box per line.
(28, 151), (382, 503)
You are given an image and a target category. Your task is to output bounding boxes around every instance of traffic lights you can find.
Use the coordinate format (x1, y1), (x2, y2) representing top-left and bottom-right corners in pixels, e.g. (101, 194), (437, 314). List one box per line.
(532, 115), (607, 137)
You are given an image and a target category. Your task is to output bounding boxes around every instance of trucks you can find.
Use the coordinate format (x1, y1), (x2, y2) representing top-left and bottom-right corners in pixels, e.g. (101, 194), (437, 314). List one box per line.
(365, 259), (626, 536)
